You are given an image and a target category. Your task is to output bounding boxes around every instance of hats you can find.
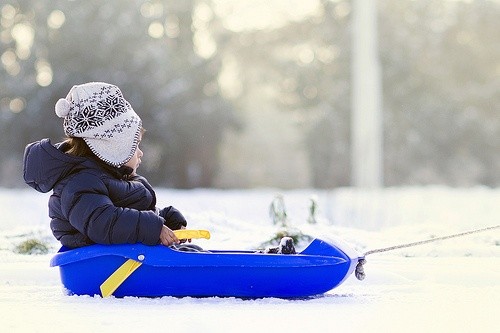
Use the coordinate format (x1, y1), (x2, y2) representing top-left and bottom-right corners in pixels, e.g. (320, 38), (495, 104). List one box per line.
(55, 82), (143, 167)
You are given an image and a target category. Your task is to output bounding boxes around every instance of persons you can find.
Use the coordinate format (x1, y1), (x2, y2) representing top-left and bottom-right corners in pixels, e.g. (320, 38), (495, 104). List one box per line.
(23, 82), (297, 255)
